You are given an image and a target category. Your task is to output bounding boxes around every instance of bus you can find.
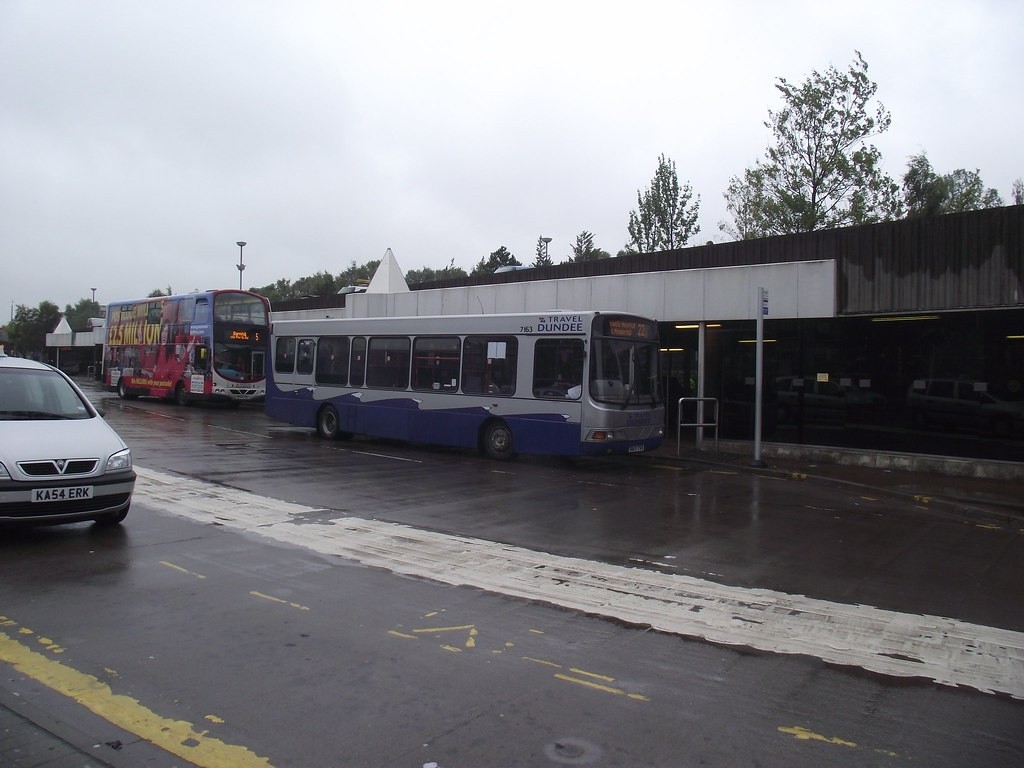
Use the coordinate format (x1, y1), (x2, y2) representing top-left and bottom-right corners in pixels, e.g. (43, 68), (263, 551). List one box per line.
(101, 290), (272, 408)
(265, 310), (667, 463)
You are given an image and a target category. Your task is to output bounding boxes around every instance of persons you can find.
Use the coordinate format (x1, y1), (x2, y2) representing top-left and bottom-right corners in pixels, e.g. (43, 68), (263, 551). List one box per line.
(255, 356), (262, 374)
(137, 319), (196, 397)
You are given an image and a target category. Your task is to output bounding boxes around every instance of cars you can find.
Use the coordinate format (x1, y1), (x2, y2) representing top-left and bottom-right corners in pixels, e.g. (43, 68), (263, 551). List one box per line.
(0, 356), (137, 528)
(906, 378), (1024, 435)
(770, 377), (867, 424)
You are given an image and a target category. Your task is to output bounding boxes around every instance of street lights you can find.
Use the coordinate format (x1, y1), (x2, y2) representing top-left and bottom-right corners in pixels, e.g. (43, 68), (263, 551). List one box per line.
(91, 287), (98, 302)
(236, 242), (247, 290)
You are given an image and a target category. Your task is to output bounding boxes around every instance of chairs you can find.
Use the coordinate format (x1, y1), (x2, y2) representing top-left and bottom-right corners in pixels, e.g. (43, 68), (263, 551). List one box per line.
(245, 319), (254, 325)
(234, 317), (243, 324)
(215, 314), (221, 321)
